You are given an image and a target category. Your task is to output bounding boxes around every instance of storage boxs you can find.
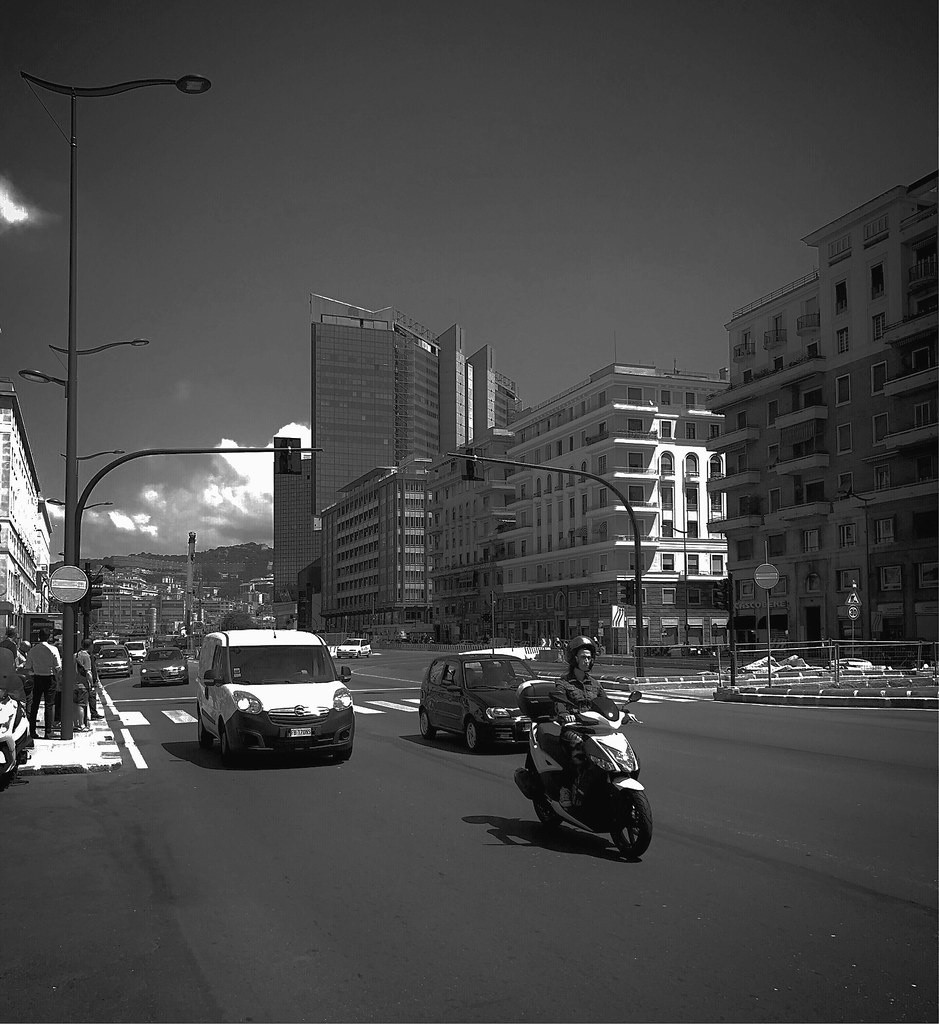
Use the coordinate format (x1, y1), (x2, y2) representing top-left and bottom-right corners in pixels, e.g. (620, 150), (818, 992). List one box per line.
(515, 680), (560, 720)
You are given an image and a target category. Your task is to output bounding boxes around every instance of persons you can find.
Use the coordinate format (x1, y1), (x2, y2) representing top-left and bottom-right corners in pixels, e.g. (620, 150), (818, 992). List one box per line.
(26, 628), (62, 739)
(0, 625), (31, 671)
(554, 635), (635, 809)
(74, 638), (104, 732)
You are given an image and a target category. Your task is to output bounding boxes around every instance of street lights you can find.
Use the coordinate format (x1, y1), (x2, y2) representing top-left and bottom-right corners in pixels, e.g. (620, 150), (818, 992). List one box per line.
(661, 524), (693, 656)
(17, 70), (215, 742)
(838, 485), (876, 661)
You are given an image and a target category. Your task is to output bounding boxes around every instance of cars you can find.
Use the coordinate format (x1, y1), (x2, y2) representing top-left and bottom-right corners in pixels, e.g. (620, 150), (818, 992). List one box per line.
(418, 653), (543, 755)
(336, 638), (372, 659)
(92, 640), (148, 678)
(139, 647), (189, 686)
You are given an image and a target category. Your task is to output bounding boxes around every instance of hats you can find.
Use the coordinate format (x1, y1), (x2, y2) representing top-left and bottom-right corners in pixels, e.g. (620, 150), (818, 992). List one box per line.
(20, 641), (31, 652)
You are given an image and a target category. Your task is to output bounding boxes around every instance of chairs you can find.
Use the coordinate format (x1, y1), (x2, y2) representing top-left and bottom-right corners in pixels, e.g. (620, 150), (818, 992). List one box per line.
(453, 667), (477, 688)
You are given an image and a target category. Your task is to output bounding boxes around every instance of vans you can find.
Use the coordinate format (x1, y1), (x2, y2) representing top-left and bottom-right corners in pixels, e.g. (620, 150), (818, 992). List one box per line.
(194, 629), (359, 765)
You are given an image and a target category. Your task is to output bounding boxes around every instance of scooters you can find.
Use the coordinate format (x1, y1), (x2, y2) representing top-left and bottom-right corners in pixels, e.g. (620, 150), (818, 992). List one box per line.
(507, 680), (655, 863)
(0, 667), (34, 785)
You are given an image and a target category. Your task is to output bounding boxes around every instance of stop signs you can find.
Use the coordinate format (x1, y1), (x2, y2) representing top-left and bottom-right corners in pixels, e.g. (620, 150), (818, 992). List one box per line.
(49, 565), (88, 604)
(754, 564), (779, 589)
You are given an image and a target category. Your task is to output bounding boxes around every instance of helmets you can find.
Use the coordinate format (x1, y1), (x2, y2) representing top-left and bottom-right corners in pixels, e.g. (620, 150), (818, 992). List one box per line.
(566, 635), (596, 669)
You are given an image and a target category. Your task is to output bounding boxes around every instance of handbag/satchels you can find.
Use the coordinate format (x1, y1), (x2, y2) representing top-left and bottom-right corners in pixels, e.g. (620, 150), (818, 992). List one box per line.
(75, 659), (86, 677)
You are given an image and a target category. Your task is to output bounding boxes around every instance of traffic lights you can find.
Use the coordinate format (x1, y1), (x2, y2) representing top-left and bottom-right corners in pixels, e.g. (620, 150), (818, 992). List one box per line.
(619, 579), (634, 606)
(712, 579), (730, 611)
(82, 572), (104, 616)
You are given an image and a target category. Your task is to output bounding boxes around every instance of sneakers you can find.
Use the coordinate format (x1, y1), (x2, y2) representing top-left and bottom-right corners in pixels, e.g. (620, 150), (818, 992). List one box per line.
(559, 787), (572, 808)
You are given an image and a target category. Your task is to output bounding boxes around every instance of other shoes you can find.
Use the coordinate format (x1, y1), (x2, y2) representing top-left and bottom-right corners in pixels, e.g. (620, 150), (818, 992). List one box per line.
(45, 733), (60, 739)
(91, 715), (105, 720)
(31, 735), (39, 738)
(73, 724), (89, 732)
